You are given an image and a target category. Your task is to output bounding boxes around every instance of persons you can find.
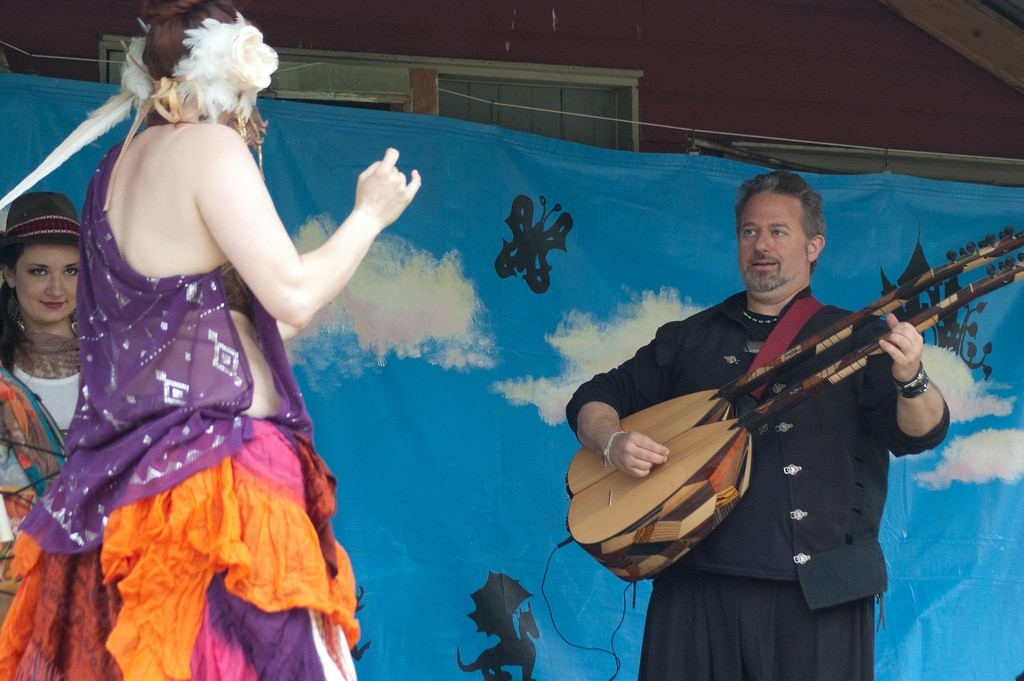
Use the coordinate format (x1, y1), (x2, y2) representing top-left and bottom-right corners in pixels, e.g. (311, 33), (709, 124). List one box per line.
(1, 0), (419, 681)
(565, 171), (949, 681)
(0, 191), (108, 630)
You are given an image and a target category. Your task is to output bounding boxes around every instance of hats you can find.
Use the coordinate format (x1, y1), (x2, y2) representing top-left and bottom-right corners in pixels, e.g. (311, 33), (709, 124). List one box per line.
(0, 192), (81, 245)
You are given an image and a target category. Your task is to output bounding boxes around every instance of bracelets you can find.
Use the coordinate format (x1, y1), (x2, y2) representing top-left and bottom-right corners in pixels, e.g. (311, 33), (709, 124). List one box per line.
(892, 360), (929, 398)
(603, 432), (627, 469)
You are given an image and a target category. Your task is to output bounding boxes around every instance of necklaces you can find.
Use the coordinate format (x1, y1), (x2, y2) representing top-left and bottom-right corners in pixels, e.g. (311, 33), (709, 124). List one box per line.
(742, 309), (777, 324)
